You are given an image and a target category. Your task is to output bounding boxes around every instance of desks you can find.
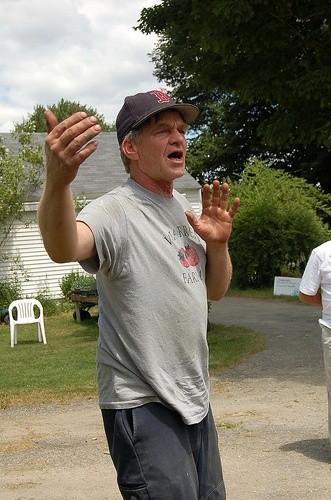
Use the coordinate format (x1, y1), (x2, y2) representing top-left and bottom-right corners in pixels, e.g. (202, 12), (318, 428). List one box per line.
(71, 294), (98, 323)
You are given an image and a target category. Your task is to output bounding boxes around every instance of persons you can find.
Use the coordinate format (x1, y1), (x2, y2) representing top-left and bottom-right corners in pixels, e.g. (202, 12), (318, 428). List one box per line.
(299, 216), (330, 462)
(37, 90), (240, 500)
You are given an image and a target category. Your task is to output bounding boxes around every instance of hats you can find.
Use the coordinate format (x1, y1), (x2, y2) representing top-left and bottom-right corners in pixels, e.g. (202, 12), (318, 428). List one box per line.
(116, 90), (200, 145)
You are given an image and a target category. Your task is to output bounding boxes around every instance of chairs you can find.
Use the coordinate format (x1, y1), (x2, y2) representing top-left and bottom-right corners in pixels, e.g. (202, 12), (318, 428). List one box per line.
(9, 299), (47, 347)
